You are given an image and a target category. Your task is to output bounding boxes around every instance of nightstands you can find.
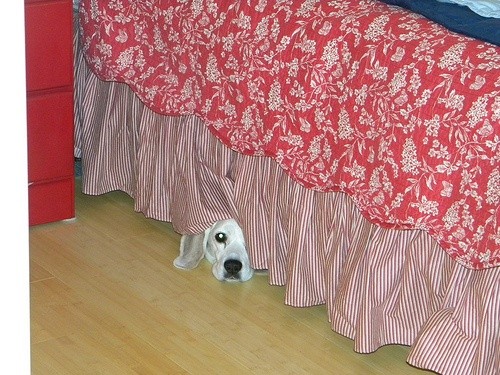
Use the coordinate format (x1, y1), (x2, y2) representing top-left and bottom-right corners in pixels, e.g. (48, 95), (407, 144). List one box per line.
(25, 1), (75, 226)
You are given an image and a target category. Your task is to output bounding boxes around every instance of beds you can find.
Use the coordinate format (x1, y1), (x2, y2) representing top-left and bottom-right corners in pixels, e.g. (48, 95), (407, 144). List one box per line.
(73, 0), (500, 375)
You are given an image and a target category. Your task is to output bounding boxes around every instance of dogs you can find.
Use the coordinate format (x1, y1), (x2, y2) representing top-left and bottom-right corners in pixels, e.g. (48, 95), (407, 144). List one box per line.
(172, 218), (256, 282)
